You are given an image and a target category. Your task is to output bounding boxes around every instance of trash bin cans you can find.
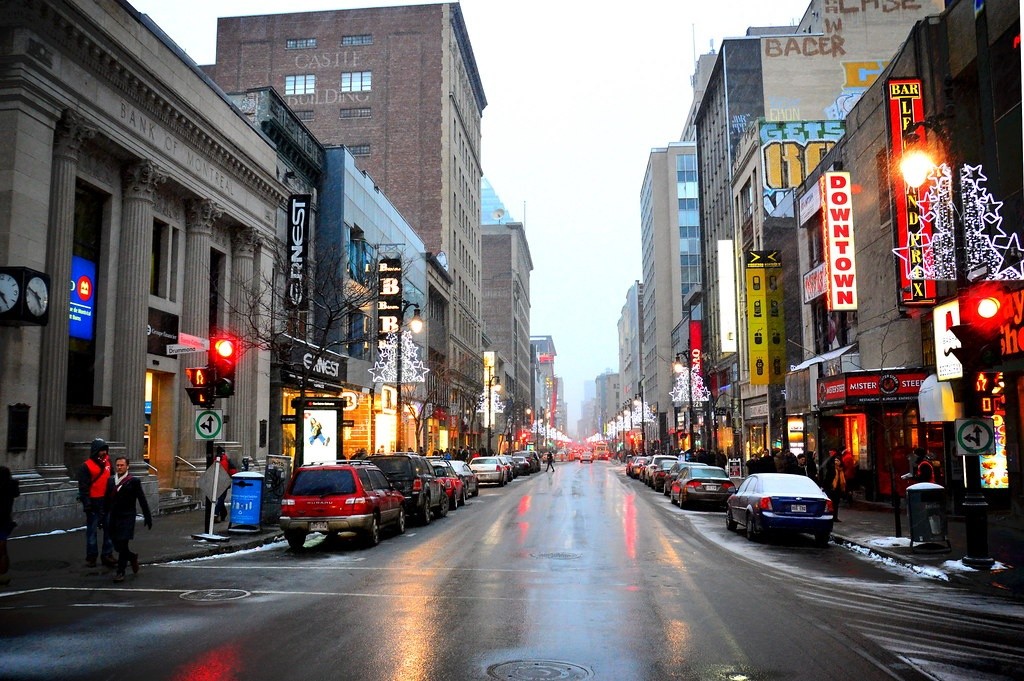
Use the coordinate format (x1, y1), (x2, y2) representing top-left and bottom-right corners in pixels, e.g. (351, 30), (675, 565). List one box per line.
(906, 483), (953, 554)
(229, 472), (264, 534)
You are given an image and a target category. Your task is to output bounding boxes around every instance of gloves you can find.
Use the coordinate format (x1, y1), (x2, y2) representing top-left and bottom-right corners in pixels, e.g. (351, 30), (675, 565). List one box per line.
(143, 517), (152, 529)
(82, 506), (90, 512)
(96, 518), (105, 529)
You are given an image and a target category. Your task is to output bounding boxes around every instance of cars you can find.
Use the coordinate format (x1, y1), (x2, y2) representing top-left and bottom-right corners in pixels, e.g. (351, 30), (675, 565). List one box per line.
(624, 454), (709, 496)
(670, 465), (737, 511)
(424, 449), (541, 523)
(725, 472), (835, 546)
(544, 449), (609, 464)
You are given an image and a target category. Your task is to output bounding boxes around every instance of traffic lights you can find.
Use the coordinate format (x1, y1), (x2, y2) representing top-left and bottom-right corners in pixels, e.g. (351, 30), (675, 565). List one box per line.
(184, 366), (212, 407)
(948, 321), (987, 375)
(977, 296), (1001, 320)
(213, 339), (236, 396)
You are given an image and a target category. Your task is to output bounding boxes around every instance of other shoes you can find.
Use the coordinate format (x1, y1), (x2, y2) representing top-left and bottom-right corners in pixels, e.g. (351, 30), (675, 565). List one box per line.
(220, 520), (227, 522)
(87, 558), (97, 567)
(832, 517), (842, 523)
(113, 570), (125, 582)
(101, 554), (118, 565)
(214, 515), (221, 524)
(132, 553), (139, 573)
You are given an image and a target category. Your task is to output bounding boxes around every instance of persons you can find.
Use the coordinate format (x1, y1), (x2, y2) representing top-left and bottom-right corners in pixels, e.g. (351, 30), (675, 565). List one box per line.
(747, 448), (817, 483)
(902, 448), (936, 483)
(669, 446), (727, 468)
(79, 438), (118, 566)
(629, 450), (633, 455)
(350, 448), (367, 460)
(213, 446), (237, 522)
(309, 418), (329, 446)
(98, 457), (152, 581)
(642, 449), (666, 456)
(819, 447), (855, 522)
(407, 444), (493, 461)
(545, 452), (554, 472)
(378, 445), (384, 452)
(0, 465), (19, 587)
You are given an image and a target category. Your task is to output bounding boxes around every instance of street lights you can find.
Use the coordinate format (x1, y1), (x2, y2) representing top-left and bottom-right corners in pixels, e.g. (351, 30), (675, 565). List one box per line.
(487, 365), (501, 456)
(898, 120), (995, 570)
(633, 386), (646, 456)
(522, 397), (531, 450)
(396, 299), (424, 453)
(623, 399), (634, 456)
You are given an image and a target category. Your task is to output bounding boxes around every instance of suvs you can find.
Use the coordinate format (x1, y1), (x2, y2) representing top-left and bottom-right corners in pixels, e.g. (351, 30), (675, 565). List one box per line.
(277, 458), (408, 550)
(359, 451), (450, 528)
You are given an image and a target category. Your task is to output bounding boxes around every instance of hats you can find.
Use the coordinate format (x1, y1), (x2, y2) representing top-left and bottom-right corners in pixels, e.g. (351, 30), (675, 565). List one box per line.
(913, 448), (926, 456)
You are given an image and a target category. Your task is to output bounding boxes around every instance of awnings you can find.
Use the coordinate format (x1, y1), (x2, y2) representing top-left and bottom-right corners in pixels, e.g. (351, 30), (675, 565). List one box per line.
(786, 344), (857, 375)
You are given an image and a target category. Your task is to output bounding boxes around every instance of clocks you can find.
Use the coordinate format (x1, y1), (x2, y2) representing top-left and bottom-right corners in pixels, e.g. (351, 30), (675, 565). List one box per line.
(13, 267), (49, 326)
(0, 266), (25, 321)
